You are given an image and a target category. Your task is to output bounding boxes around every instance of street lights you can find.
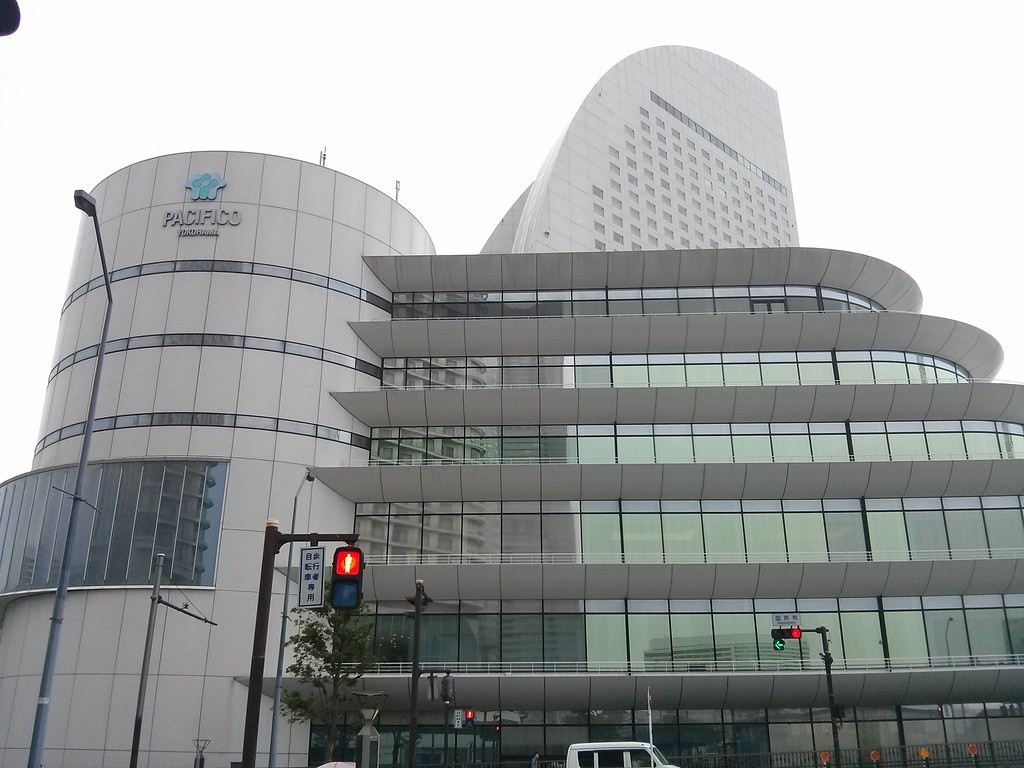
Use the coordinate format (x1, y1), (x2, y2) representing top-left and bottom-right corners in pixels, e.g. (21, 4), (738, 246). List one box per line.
(945, 618), (953, 665)
(27, 190), (113, 768)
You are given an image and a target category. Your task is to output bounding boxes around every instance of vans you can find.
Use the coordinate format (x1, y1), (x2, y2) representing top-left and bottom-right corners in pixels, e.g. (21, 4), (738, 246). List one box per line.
(566, 742), (682, 768)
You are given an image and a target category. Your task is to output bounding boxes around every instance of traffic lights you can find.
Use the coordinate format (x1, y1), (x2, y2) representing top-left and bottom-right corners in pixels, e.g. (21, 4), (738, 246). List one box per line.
(466, 710), (474, 728)
(771, 626), (802, 650)
(495, 723), (501, 734)
(330, 547), (364, 609)
